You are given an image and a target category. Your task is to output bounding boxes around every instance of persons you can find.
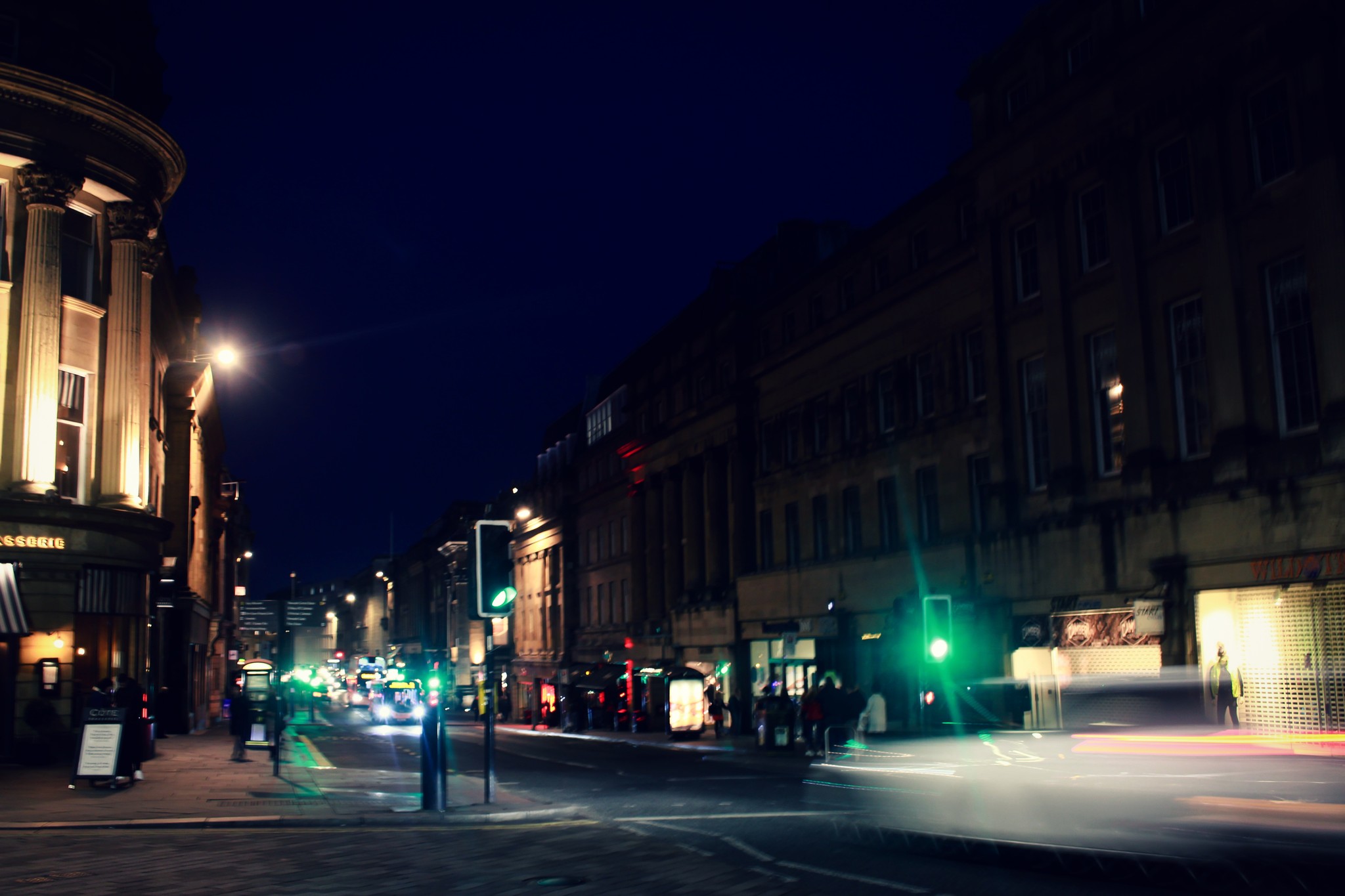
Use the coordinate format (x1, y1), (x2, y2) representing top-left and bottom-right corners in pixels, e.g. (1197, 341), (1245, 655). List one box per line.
(1209, 645), (1245, 729)
(112, 675), (148, 782)
(861, 685), (888, 755)
(227, 684), (253, 760)
(283, 667), (868, 761)
(68, 677), (115, 789)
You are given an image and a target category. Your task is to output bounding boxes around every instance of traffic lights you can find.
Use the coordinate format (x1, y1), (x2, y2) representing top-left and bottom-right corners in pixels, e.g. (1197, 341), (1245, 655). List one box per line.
(426, 651), (443, 707)
(923, 595), (953, 662)
(476, 519), (515, 618)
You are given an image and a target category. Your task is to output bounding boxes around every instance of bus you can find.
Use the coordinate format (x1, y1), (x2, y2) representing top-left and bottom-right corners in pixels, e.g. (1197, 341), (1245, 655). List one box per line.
(347, 654), (387, 706)
(370, 679), (421, 723)
